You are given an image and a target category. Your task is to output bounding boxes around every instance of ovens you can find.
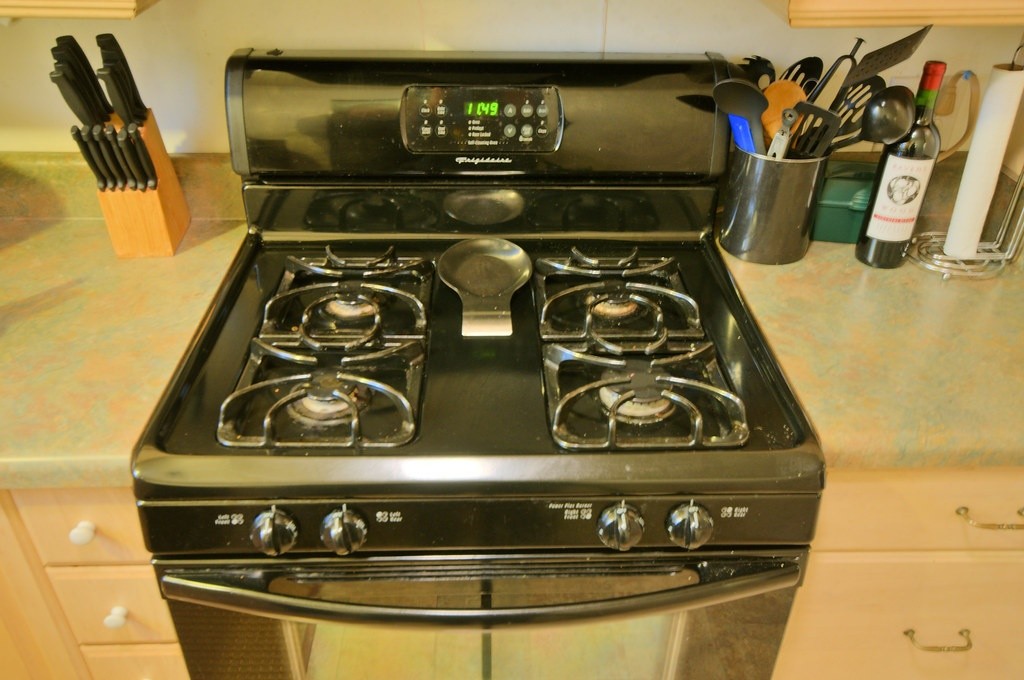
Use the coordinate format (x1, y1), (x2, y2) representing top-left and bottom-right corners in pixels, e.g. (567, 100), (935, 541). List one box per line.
(145, 559), (800, 680)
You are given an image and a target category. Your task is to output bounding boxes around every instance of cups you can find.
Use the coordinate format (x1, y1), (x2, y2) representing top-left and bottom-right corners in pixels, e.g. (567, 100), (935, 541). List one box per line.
(718, 143), (828, 263)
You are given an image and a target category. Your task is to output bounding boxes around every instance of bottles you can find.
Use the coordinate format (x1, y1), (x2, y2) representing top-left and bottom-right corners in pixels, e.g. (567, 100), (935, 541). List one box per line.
(853, 62), (948, 269)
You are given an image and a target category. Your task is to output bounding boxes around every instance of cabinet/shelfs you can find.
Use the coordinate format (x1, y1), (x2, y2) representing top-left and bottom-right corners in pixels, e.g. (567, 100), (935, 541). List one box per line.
(1, 486), (191, 680)
(770, 469), (1023, 680)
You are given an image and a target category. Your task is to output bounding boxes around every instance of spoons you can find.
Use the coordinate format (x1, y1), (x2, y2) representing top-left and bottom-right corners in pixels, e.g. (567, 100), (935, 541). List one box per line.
(713, 80), (765, 157)
(828, 85), (916, 153)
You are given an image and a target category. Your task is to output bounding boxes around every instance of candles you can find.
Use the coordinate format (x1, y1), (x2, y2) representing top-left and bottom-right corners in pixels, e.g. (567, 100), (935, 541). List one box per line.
(944, 43), (1024, 257)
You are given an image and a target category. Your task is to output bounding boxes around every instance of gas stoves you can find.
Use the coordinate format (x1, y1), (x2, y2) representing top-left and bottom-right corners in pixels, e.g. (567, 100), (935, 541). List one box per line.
(132, 45), (825, 553)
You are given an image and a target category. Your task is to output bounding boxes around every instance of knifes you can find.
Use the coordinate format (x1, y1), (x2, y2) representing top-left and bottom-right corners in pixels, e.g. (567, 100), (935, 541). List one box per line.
(49, 34), (156, 190)
